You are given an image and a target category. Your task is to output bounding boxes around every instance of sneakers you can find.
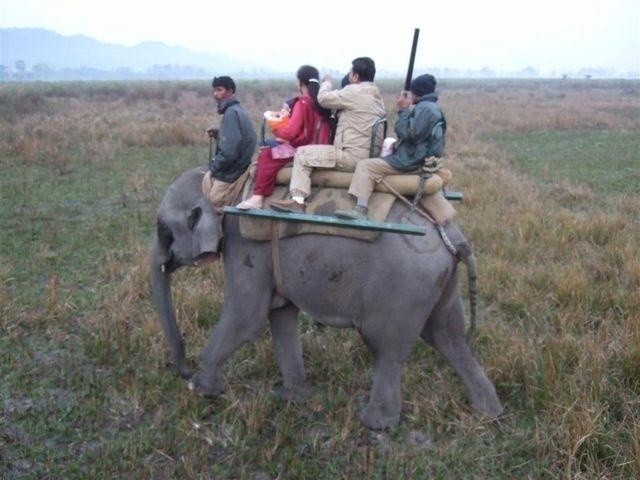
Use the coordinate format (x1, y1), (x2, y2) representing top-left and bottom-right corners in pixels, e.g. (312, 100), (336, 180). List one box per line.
(236, 201), (264, 208)
(335, 209), (368, 220)
(270, 199), (305, 213)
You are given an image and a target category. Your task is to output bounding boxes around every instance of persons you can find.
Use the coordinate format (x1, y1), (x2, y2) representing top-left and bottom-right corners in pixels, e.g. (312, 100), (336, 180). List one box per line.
(234, 64), (331, 212)
(200, 75), (258, 215)
(266, 56), (388, 215)
(331, 73), (446, 221)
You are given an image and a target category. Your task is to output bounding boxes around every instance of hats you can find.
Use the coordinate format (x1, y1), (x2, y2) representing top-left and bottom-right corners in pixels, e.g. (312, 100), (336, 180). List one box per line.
(410, 74), (435, 95)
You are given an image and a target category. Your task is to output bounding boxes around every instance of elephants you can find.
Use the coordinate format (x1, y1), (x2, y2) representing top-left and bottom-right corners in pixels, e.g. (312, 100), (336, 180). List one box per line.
(145, 160), (505, 432)
